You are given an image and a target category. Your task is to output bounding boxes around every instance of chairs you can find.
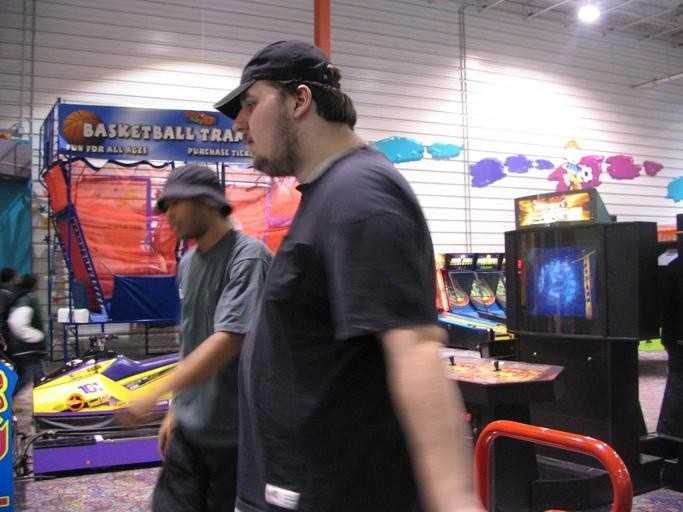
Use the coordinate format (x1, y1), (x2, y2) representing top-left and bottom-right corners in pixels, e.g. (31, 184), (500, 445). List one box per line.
(100, 274), (182, 355)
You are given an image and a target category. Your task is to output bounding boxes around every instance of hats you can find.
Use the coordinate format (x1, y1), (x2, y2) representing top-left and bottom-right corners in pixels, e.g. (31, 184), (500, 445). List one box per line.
(214, 40), (339, 120)
(157, 166), (231, 216)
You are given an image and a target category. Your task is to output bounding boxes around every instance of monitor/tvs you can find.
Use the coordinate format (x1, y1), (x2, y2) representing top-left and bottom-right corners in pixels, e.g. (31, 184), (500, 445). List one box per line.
(504, 222), (660, 342)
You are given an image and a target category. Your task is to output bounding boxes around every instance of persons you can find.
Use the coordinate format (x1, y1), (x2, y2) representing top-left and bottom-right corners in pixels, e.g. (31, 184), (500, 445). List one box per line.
(5, 274), (48, 465)
(1, 268), (18, 372)
(214, 41), (486, 509)
(118, 165), (277, 509)
